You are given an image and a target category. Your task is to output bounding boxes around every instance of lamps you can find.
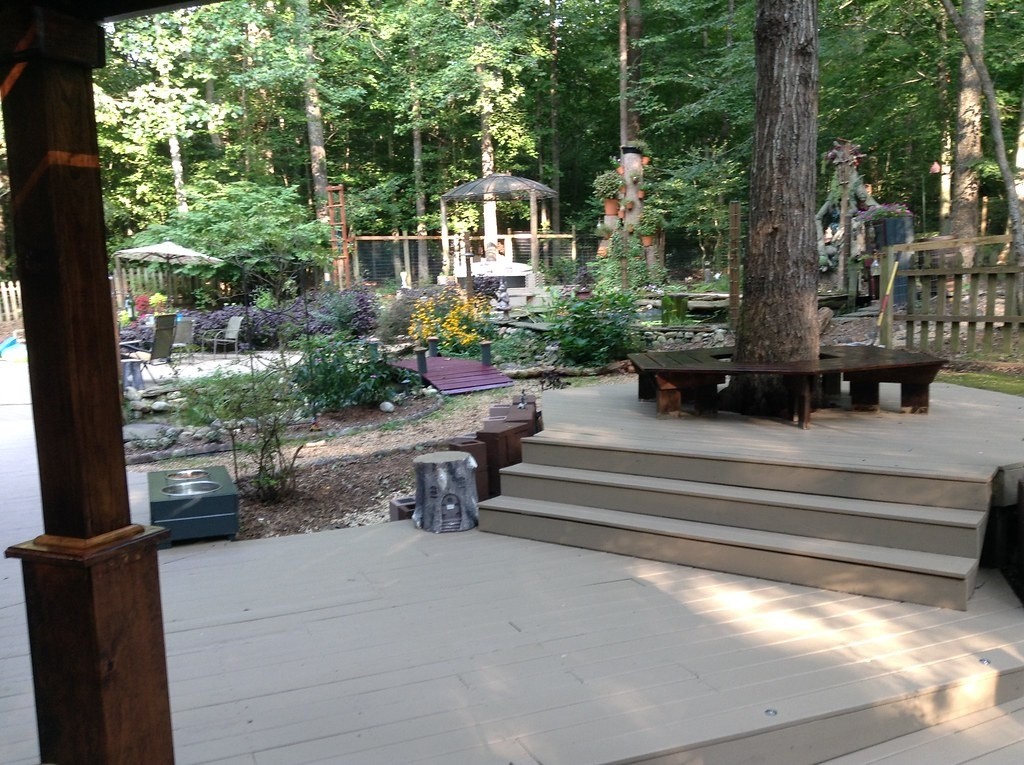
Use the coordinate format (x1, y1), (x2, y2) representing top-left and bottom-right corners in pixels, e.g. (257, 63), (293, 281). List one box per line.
(870, 251), (886, 276)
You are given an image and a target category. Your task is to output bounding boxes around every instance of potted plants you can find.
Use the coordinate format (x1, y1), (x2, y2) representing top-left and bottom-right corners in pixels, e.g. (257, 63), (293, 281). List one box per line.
(590, 139), (662, 247)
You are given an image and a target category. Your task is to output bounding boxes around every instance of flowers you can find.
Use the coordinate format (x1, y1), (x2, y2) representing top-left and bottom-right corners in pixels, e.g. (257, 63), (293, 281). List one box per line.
(854, 202), (914, 225)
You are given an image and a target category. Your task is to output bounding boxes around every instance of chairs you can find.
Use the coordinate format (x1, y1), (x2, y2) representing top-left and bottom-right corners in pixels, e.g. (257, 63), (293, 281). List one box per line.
(123, 313), (178, 385)
(201, 315), (245, 361)
(169, 320), (193, 363)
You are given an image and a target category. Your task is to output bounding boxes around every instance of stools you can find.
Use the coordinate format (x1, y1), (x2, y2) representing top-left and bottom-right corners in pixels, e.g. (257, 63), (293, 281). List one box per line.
(121, 360), (147, 391)
(412, 450), (479, 535)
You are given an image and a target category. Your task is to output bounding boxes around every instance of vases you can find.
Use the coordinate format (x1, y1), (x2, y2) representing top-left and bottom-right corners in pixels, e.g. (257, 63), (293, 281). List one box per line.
(864, 217), (959, 307)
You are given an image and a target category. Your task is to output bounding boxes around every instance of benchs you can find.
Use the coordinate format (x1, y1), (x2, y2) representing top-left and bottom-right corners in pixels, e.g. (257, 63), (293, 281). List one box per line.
(455, 259), (534, 289)
(627, 346), (949, 431)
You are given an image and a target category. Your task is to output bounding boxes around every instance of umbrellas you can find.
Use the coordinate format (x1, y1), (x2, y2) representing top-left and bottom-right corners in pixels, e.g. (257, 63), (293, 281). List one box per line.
(441, 172), (559, 205)
(111, 241), (224, 309)
